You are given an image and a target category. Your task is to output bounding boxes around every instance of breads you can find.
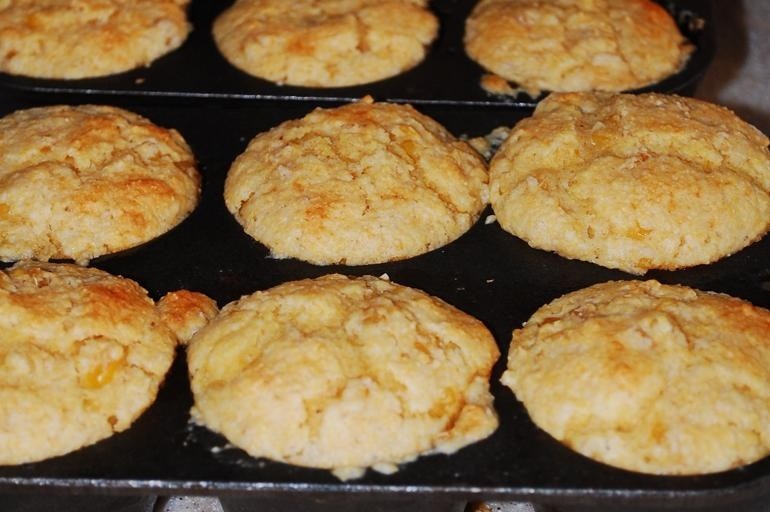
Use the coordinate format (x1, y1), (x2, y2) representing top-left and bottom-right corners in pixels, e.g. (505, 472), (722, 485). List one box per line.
(0, 0), (190, 80)
(223, 94), (491, 267)
(0, 104), (201, 263)
(213, 1), (440, 88)
(501, 280), (770, 477)
(463, 1), (695, 93)
(490, 92), (770, 275)
(0, 260), (217, 466)
(187, 274), (500, 481)
